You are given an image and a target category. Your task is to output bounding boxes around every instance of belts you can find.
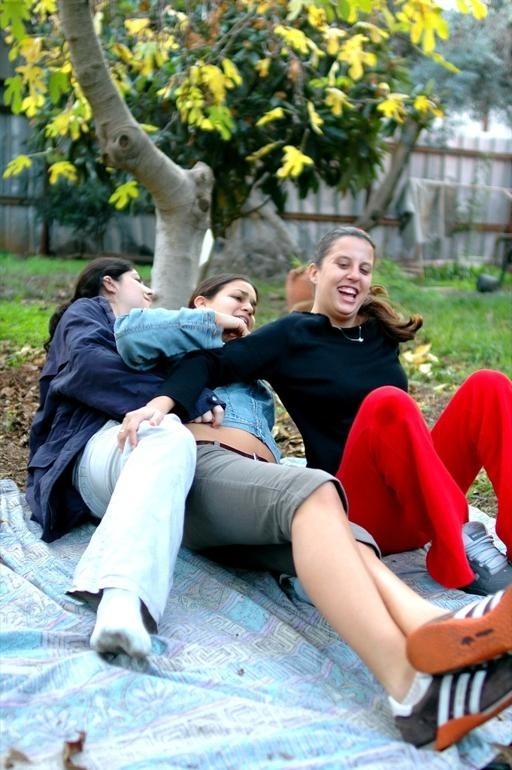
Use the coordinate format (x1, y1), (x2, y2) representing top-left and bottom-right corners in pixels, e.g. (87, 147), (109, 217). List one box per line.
(195, 438), (273, 464)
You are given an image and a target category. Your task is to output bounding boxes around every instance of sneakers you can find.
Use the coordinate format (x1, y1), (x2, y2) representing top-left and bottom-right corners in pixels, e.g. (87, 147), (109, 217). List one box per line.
(444, 515), (512, 599)
(386, 653), (512, 754)
(403, 581), (512, 676)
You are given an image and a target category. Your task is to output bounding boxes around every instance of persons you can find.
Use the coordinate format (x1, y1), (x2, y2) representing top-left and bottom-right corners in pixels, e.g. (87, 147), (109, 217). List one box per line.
(25, 256), (229, 657)
(117, 224), (511, 603)
(109, 271), (512, 757)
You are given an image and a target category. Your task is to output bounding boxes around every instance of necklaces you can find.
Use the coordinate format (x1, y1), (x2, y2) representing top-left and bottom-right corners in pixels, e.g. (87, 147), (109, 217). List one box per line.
(332, 318), (363, 344)
